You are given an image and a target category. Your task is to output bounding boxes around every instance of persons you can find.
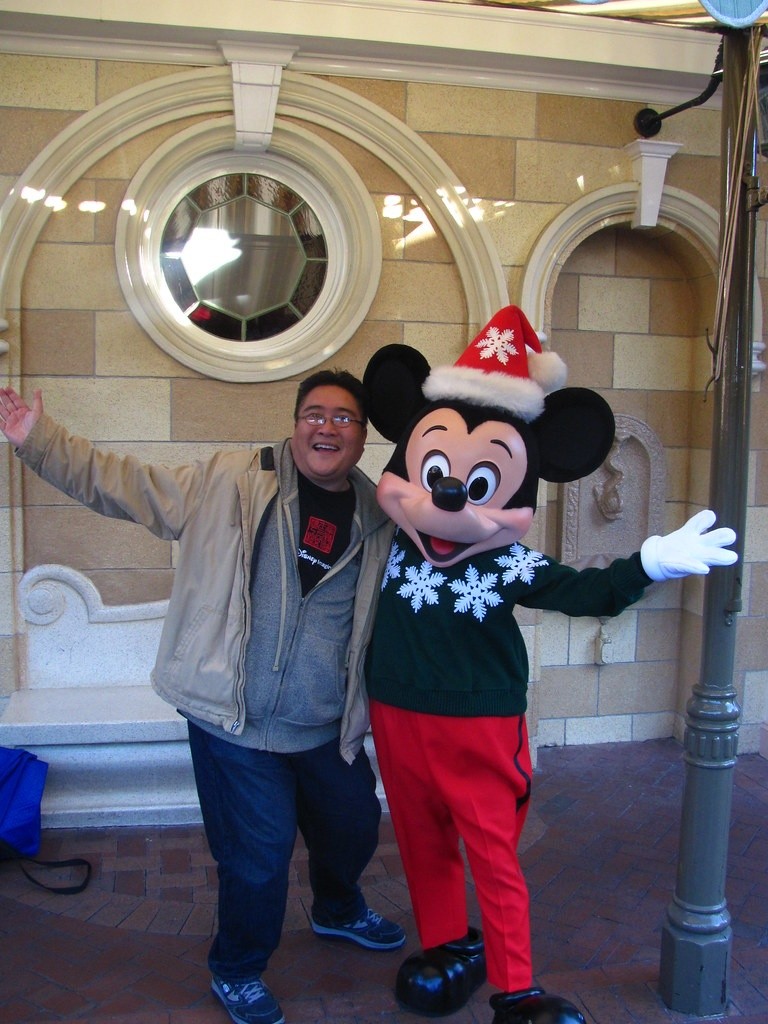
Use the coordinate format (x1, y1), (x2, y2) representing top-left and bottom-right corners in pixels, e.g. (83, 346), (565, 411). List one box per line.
(0, 365), (405, 1024)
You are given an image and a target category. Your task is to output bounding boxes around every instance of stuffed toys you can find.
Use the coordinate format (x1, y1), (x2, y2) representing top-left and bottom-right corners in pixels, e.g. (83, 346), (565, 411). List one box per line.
(359, 305), (739, 1023)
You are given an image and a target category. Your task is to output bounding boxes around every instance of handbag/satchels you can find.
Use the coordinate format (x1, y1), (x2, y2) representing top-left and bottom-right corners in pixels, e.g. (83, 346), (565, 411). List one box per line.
(0, 746), (50, 858)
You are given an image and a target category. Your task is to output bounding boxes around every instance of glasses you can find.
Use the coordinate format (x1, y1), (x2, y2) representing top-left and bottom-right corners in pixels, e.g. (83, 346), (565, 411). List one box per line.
(299, 412), (364, 428)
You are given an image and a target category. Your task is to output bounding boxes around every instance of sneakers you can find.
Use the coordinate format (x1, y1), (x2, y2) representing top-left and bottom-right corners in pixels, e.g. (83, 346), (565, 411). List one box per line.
(310, 906), (406, 951)
(210, 968), (285, 1024)
